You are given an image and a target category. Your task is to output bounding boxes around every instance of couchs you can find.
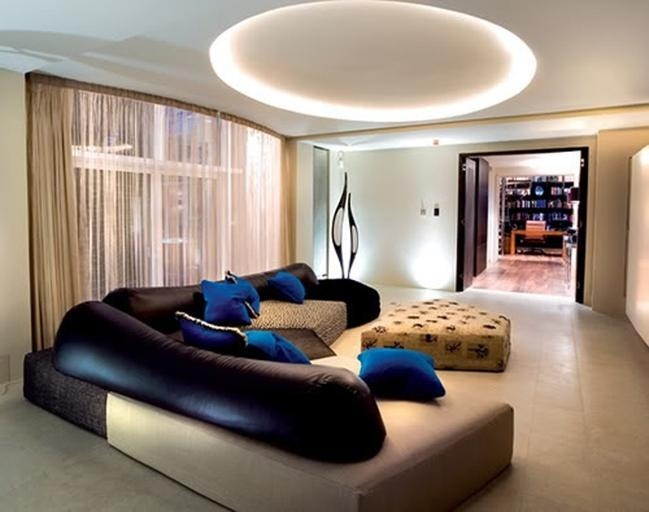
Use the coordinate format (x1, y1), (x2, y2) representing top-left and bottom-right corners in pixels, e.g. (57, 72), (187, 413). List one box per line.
(94, 257), (384, 359)
(18, 298), (519, 512)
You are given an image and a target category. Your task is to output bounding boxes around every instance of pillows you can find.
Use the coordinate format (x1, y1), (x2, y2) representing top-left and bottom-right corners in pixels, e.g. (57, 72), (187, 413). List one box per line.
(172, 306), (253, 361)
(196, 274), (255, 328)
(242, 324), (318, 367)
(353, 344), (447, 404)
(265, 268), (306, 307)
(220, 269), (263, 319)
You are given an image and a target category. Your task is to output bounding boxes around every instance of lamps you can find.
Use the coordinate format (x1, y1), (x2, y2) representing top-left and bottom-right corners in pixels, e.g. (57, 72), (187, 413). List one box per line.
(330, 170), (360, 280)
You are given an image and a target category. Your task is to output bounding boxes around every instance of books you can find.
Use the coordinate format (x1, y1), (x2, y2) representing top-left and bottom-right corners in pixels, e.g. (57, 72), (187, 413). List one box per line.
(499, 174), (572, 233)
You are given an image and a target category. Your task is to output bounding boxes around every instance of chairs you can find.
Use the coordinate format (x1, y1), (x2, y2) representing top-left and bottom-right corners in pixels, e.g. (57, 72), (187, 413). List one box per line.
(519, 218), (548, 257)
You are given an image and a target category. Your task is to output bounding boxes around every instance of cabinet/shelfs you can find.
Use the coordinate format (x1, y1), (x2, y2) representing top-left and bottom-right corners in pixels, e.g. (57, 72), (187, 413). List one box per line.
(497, 176), (574, 256)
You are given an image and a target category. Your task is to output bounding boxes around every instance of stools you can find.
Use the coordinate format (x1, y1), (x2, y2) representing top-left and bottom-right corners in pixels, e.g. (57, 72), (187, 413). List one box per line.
(354, 290), (514, 377)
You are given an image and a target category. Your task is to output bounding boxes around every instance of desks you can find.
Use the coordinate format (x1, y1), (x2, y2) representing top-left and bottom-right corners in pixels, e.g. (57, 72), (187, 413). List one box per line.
(508, 227), (570, 257)
